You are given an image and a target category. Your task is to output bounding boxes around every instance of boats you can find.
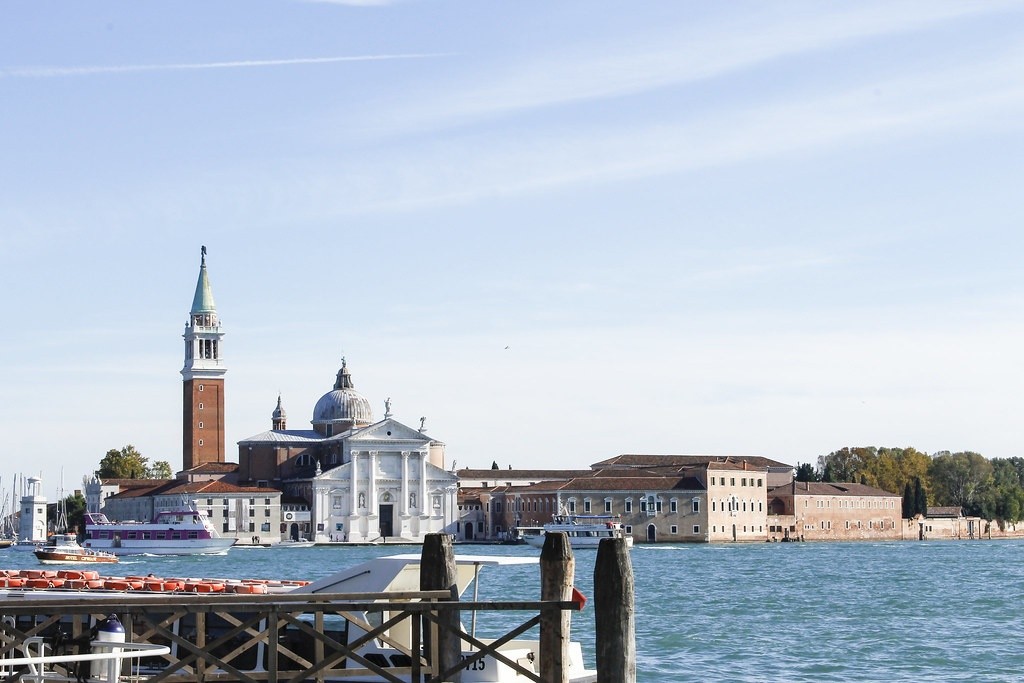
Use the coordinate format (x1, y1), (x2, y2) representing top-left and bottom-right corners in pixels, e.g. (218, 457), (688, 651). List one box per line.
(31, 534), (119, 565)
(0, 553), (598, 682)
(81, 490), (240, 555)
(522, 513), (635, 549)
